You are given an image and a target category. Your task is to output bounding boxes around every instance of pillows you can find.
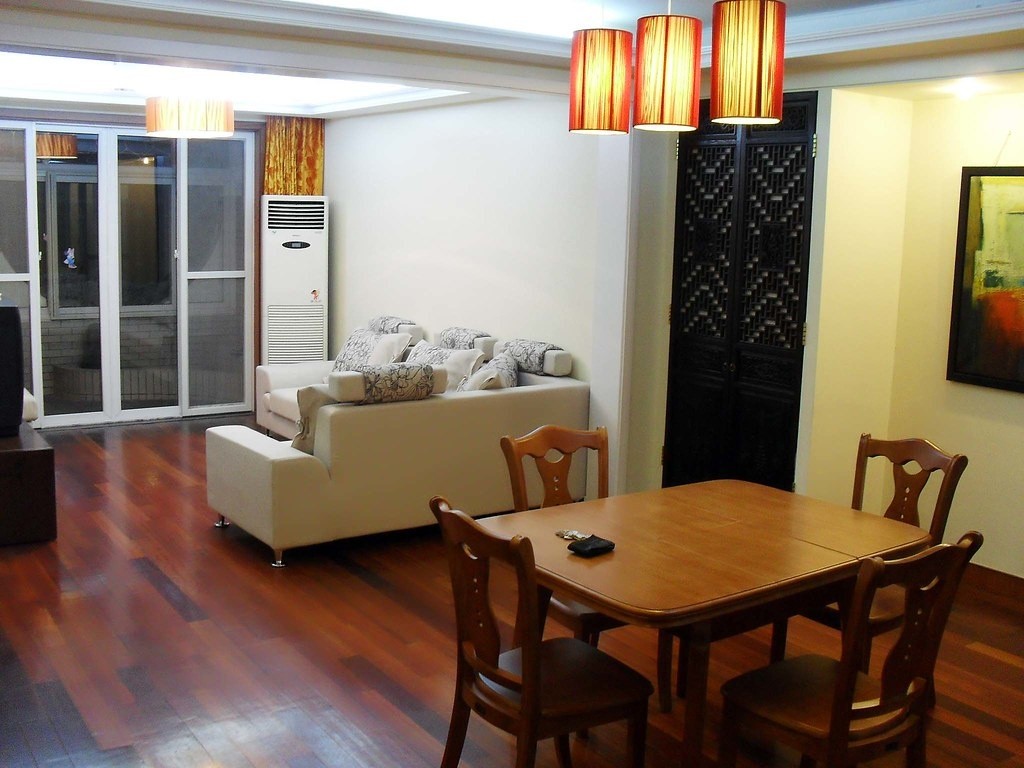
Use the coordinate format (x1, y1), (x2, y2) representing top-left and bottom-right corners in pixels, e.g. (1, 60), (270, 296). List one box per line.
(292, 384), (339, 454)
(405, 339), (486, 394)
(456, 347), (518, 392)
(324, 328), (413, 382)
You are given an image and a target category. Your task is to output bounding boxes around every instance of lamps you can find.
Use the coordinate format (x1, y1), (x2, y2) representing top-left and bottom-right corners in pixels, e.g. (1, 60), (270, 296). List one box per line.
(146, 95), (234, 138)
(36, 133), (78, 159)
(632, 14), (702, 131)
(710, 0), (786, 124)
(569, 28), (633, 135)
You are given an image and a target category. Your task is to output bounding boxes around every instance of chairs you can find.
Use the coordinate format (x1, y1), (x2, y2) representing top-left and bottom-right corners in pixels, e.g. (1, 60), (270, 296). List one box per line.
(499, 424), (673, 739)
(770, 432), (969, 708)
(428, 495), (655, 768)
(719, 530), (985, 768)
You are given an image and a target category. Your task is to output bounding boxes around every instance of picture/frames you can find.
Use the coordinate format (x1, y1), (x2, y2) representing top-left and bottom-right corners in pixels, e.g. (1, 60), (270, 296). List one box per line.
(945, 166), (1024, 393)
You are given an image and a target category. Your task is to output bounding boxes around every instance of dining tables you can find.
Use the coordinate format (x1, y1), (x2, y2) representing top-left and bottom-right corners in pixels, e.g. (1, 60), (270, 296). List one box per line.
(474, 479), (934, 768)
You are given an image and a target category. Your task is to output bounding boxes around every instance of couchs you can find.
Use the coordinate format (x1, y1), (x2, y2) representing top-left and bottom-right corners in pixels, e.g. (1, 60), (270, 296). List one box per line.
(205, 315), (590, 568)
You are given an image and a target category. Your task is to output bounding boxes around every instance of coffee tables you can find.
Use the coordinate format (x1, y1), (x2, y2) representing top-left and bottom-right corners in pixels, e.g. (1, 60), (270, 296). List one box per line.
(0, 418), (57, 545)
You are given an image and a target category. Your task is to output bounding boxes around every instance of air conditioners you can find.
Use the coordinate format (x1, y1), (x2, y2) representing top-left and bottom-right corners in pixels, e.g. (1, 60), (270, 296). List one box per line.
(260, 195), (329, 365)
